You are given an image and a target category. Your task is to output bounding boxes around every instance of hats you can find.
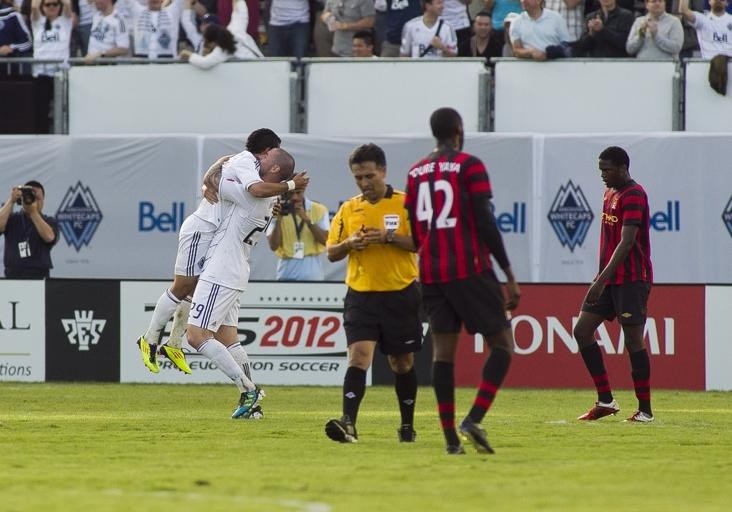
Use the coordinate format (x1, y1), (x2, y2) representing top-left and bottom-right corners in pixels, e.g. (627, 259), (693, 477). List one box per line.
(195, 13), (219, 24)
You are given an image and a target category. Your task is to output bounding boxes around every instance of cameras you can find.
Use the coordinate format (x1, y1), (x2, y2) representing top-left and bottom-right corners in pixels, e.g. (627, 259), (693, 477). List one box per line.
(278, 198), (294, 214)
(15, 185), (35, 204)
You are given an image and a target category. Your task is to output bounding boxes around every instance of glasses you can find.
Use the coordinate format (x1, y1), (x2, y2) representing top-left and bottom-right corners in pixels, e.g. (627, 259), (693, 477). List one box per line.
(43, 1), (61, 8)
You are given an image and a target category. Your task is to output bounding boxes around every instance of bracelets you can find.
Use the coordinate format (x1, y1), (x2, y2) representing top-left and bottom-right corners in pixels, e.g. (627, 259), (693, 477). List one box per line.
(285, 180), (296, 193)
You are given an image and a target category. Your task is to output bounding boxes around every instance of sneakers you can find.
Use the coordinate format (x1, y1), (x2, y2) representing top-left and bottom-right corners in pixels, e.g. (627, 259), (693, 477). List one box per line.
(622, 409), (654, 424)
(325, 418), (358, 444)
(578, 398), (620, 422)
(136, 333), (160, 374)
(231, 385), (266, 419)
(446, 443), (465, 454)
(399, 423), (416, 442)
(158, 342), (193, 375)
(459, 416), (495, 454)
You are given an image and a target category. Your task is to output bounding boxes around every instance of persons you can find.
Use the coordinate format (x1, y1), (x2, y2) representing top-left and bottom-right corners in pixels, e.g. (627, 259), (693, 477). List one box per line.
(574, 147), (654, 423)
(324, 143), (423, 442)
(322, 0), (732, 75)
(1, 1), (327, 111)
(402, 107), (522, 454)
(136, 127), (311, 375)
(186, 149), (295, 419)
(0, 181), (61, 279)
(268, 172), (330, 281)
